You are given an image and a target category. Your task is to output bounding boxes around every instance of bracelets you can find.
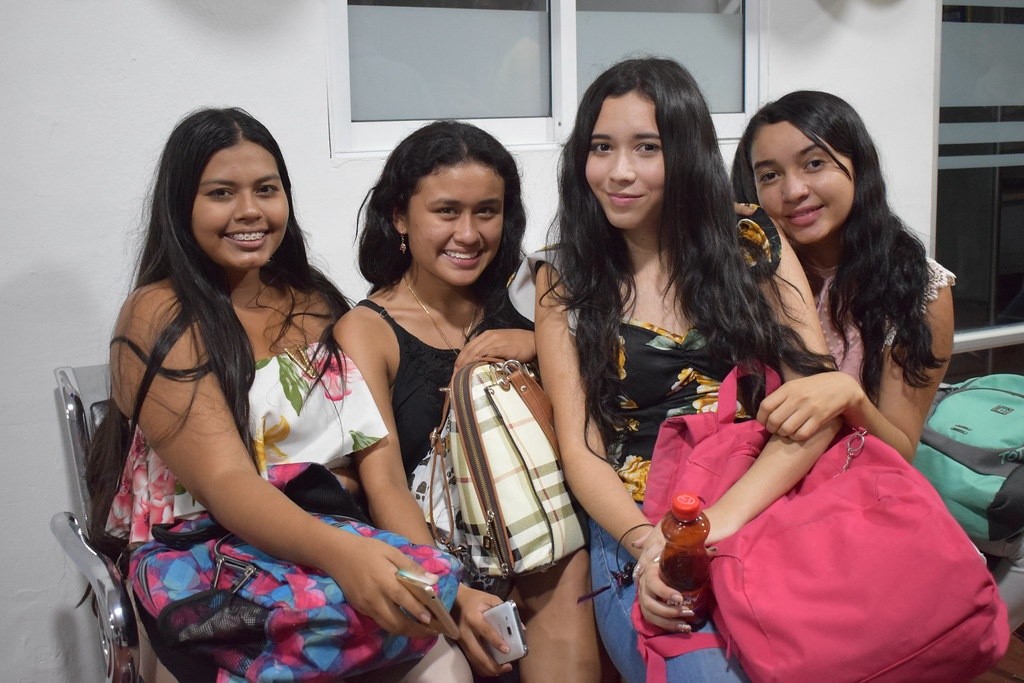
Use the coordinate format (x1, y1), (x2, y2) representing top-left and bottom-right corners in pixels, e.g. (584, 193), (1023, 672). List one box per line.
(611, 523), (655, 587)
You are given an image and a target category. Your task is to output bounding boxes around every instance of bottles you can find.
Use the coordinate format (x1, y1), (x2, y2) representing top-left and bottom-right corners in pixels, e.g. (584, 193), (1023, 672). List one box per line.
(656, 494), (712, 632)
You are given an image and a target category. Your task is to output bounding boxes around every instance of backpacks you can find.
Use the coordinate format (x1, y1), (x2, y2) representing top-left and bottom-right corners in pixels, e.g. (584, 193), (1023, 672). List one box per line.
(913, 372), (1024, 541)
(632, 361), (1010, 682)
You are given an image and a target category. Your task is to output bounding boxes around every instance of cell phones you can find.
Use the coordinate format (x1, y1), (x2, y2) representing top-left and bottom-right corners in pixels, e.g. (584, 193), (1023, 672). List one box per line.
(395, 574), (461, 640)
(480, 600), (528, 666)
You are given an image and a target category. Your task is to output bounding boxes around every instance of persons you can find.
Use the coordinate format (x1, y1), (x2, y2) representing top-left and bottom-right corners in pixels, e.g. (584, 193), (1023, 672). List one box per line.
(334, 120), (601, 682)
(84, 107), (474, 683)
(508, 57), (846, 683)
(730, 90), (1023, 636)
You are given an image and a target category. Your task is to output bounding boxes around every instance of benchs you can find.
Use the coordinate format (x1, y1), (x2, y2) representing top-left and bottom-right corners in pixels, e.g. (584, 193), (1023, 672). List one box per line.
(48, 362), (140, 682)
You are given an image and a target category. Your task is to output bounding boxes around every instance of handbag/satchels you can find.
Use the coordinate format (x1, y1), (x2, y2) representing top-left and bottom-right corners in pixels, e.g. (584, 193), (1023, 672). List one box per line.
(121, 462), (465, 683)
(429, 356), (591, 578)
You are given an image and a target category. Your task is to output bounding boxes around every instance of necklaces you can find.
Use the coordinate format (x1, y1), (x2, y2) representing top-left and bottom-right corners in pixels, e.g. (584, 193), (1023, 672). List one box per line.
(404, 273), (478, 357)
